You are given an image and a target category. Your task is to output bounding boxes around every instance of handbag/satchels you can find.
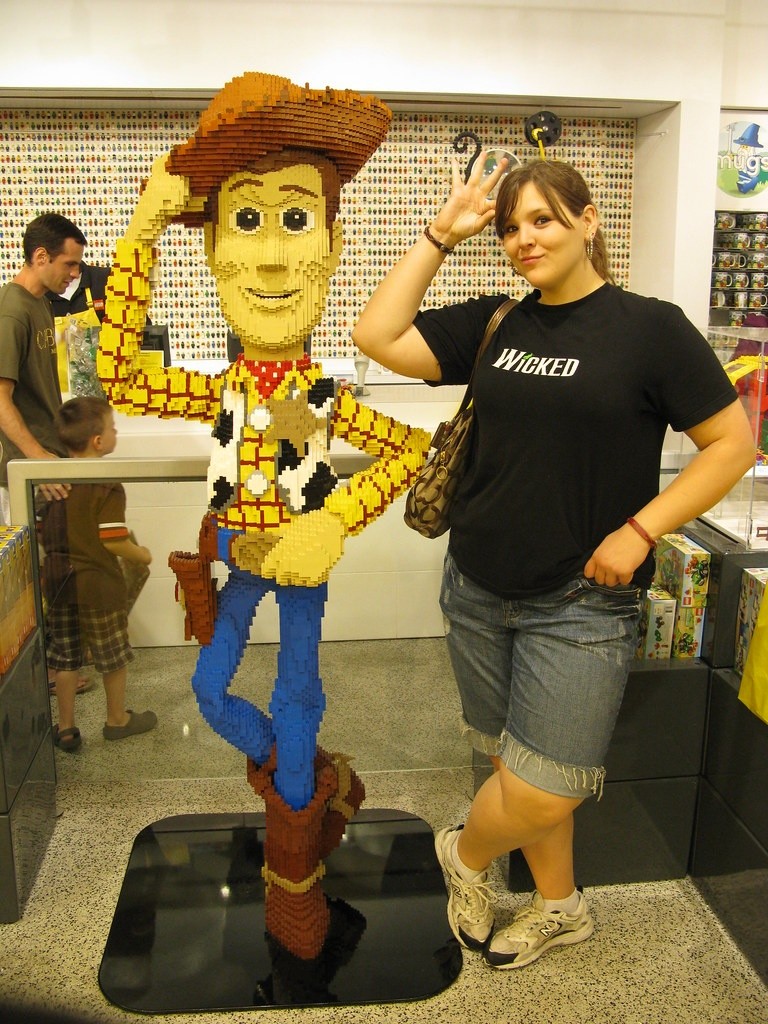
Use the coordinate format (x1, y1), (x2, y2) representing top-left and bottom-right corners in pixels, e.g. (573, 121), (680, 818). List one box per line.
(403, 299), (521, 540)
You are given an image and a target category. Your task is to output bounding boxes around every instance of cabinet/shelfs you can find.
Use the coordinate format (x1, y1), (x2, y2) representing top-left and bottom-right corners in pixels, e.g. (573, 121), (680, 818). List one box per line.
(715, 210), (768, 313)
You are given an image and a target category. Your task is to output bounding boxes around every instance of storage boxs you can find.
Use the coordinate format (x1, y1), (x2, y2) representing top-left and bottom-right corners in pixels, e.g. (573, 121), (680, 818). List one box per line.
(734, 567), (768, 679)
(678, 326), (768, 552)
(0, 525), (39, 674)
(672, 597), (706, 659)
(633, 582), (677, 660)
(653, 534), (712, 607)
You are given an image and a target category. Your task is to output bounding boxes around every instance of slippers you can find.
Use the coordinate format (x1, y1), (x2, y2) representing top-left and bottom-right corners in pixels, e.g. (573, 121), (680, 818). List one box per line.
(48, 675), (94, 695)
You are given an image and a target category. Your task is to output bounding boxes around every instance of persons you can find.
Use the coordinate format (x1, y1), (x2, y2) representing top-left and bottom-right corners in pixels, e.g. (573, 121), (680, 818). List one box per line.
(352, 142), (756, 973)
(95, 71), (433, 967)
(0, 214), (157, 750)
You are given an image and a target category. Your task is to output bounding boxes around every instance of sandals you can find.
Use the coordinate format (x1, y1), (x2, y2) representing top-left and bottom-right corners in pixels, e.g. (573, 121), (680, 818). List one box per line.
(53, 723), (82, 752)
(101, 709), (158, 740)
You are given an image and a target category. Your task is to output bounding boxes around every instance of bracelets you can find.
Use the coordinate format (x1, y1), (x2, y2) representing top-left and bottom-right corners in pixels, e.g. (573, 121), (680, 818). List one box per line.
(424, 226), (454, 254)
(626, 517), (657, 547)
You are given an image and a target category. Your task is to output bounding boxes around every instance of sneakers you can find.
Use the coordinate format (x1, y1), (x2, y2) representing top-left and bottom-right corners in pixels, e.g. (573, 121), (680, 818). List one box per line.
(434, 824), (497, 951)
(482, 887), (594, 970)
